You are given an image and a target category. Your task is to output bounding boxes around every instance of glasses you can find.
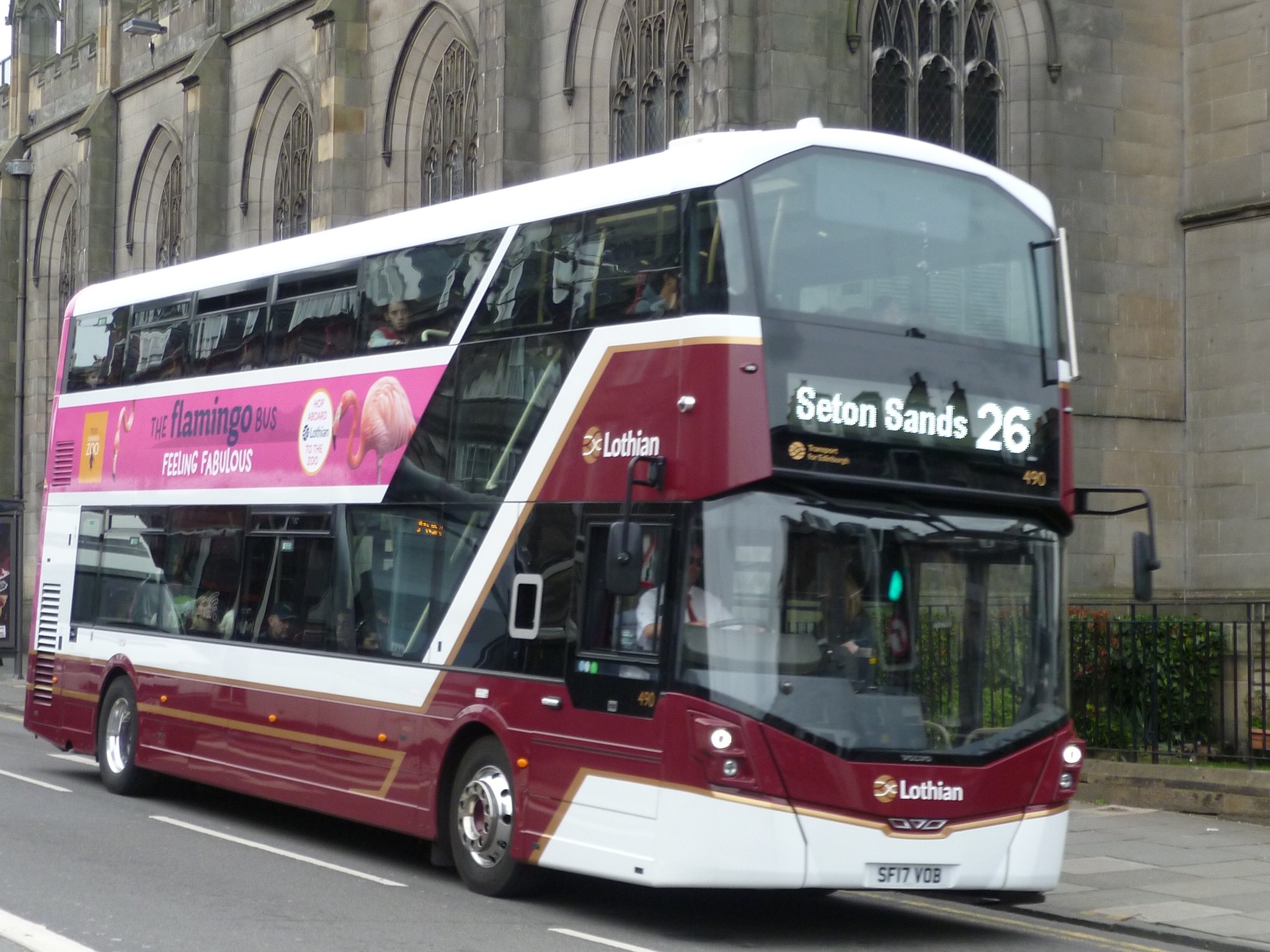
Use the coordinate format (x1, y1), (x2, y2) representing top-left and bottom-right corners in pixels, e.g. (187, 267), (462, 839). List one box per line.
(689, 556), (703, 567)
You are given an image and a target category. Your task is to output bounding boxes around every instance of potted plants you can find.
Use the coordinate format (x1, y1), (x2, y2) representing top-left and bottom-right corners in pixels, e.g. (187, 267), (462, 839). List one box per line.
(1245, 715), (1270, 750)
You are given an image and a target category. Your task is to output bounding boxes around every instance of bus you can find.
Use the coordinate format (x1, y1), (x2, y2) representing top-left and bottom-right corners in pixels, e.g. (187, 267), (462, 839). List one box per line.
(22, 116), (1167, 897)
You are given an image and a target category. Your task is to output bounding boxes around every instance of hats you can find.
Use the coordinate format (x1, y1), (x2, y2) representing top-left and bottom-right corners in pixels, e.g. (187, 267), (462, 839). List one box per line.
(269, 601), (298, 620)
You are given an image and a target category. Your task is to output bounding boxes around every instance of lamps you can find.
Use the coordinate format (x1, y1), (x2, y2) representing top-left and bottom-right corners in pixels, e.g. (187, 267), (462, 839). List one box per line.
(123, 18), (167, 36)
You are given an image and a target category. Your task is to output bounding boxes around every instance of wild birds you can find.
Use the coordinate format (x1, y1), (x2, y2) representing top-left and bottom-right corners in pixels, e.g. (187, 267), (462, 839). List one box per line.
(332, 376), (418, 485)
(112, 399), (136, 480)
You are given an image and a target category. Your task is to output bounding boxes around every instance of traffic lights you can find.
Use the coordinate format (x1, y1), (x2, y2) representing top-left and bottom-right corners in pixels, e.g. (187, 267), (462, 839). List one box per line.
(874, 543), (917, 671)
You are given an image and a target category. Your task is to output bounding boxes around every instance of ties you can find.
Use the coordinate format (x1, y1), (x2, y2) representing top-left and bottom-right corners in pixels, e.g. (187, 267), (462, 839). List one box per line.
(685, 595), (700, 622)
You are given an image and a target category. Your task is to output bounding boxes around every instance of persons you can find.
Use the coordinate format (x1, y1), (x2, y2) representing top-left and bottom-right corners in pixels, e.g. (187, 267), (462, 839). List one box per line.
(624, 252), (679, 320)
(636, 540), (766, 655)
(130, 549), (406, 651)
(807, 574), (881, 694)
(71, 292), (470, 392)
